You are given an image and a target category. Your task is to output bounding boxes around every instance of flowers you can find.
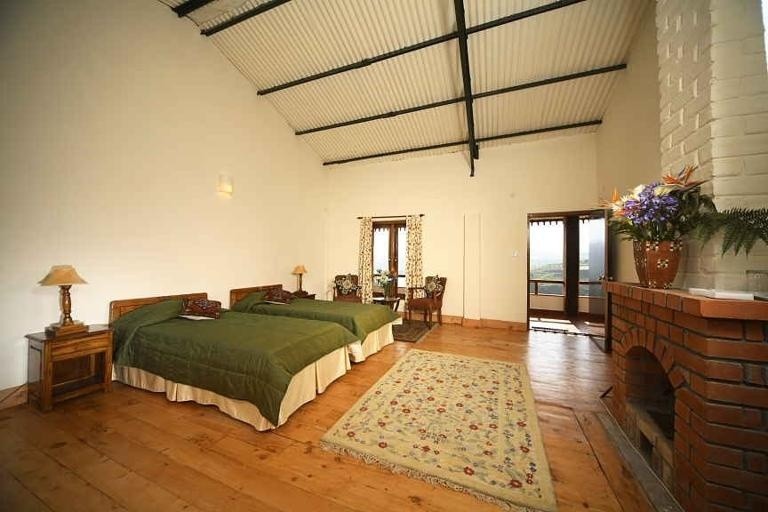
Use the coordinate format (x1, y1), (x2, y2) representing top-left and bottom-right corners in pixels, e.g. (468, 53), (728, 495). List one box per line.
(375, 269), (398, 297)
(597, 162), (718, 240)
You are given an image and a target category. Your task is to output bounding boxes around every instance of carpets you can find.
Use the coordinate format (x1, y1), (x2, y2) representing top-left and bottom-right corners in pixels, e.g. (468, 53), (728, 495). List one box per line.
(321, 348), (559, 512)
(392, 319), (436, 342)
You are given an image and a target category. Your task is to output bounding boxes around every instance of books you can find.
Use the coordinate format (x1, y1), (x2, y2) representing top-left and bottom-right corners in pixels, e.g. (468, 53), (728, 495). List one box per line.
(753, 291), (768, 302)
(688, 287), (755, 302)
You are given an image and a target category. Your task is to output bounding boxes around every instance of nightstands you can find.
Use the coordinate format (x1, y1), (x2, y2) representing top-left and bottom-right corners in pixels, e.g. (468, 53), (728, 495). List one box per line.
(24, 325), (114, 413)
(302, 294), (316, 299)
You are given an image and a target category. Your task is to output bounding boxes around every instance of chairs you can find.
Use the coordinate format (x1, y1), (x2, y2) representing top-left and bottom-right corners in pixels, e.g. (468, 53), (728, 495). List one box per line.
(333, 275), (362, 304)
(407, 276), (447, 330)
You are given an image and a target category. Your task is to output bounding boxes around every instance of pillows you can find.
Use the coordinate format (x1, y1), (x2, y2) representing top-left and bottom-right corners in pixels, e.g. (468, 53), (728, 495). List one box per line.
(264, 288), (296, 304)
(333, 273), (362, 297)
(180, 295), (221, 318)
(424, 274), (444, 298)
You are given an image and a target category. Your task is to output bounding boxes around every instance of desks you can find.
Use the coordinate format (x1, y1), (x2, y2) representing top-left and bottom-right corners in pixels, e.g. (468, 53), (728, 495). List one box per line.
(373, 298), (401, 312)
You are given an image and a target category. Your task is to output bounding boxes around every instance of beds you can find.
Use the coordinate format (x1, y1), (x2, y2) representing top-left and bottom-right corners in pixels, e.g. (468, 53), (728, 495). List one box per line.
(109, 292), (343, 432)
(230, 285), (388, 358)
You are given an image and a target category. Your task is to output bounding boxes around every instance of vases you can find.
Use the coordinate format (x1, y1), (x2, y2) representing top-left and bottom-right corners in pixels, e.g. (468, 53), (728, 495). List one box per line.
(633, 239), (683, 290)
(384, 285), (391, 297)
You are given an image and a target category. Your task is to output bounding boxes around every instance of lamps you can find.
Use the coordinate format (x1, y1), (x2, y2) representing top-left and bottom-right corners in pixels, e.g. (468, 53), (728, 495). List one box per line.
(39, 266), (89, 337)
(291, 265), (308, 296)
